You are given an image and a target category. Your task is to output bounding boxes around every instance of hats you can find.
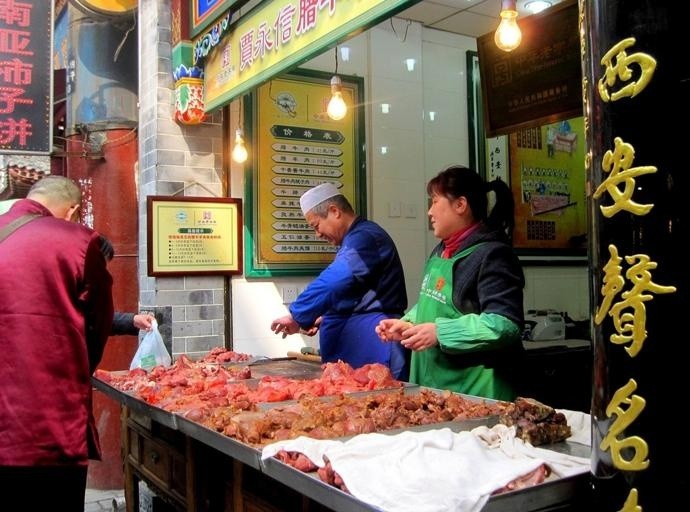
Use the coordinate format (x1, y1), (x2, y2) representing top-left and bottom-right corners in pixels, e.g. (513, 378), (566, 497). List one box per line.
(298, 182), (343, 217)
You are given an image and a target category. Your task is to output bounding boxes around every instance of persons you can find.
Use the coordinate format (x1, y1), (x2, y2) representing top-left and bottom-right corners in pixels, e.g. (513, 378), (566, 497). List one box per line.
(373, 164), (525, 405)
(0, 175), (115, 512)
(270, 182), (410, 382)
(90, 230), (157, 334)
